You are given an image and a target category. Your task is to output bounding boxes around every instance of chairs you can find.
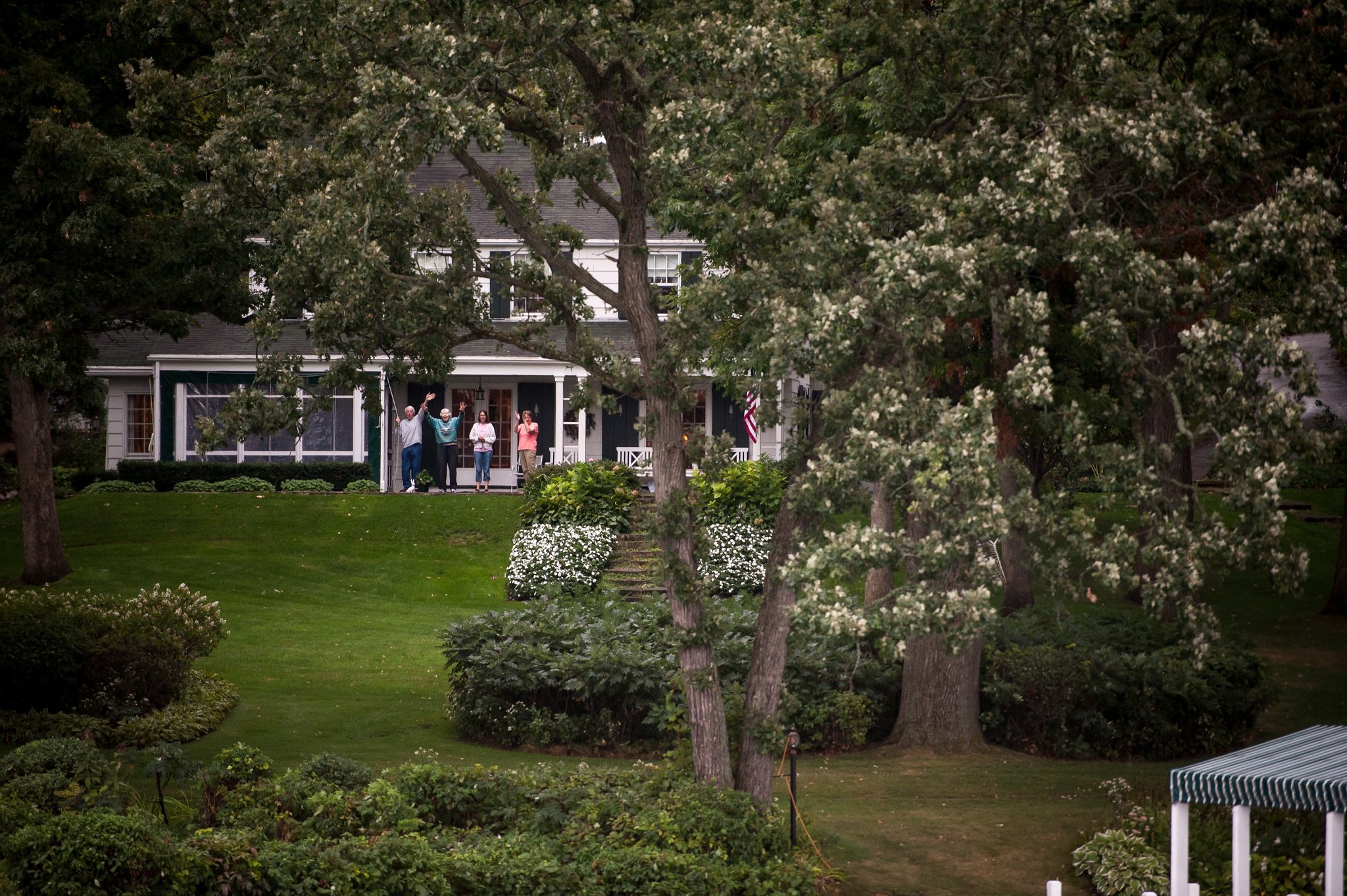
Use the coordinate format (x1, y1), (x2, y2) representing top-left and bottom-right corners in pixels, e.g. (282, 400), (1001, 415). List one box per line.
(511, 454), (544, 493)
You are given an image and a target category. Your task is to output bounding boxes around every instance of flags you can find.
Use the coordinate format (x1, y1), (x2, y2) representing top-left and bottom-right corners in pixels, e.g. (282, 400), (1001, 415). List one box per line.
(744, 367), (757, 444)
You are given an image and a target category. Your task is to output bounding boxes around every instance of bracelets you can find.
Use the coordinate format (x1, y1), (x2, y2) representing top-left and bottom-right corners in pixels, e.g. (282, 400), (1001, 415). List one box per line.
(524, 420), (526, 422)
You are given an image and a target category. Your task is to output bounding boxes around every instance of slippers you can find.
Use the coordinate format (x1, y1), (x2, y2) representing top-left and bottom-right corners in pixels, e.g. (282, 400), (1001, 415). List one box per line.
(485, 489), (489, 493)
(474, 489), (479, 493)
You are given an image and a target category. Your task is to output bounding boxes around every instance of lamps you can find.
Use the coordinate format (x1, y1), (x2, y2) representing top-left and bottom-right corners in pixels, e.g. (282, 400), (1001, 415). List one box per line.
(475, 375), (485, 400)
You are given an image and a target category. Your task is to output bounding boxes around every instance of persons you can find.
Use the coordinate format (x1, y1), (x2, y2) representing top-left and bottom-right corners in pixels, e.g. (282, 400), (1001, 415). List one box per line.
(469, 410), (496, 493)
(420, 402), (466, 493)
(396, 392), (435, 492)
(514, 411), (539, 494)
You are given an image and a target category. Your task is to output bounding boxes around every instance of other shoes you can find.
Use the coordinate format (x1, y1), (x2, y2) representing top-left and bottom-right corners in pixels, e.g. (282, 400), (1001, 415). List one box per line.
(399, 488), (407, 493)
(439, 489), (445, 493)
(450, 489), (456, 494)
